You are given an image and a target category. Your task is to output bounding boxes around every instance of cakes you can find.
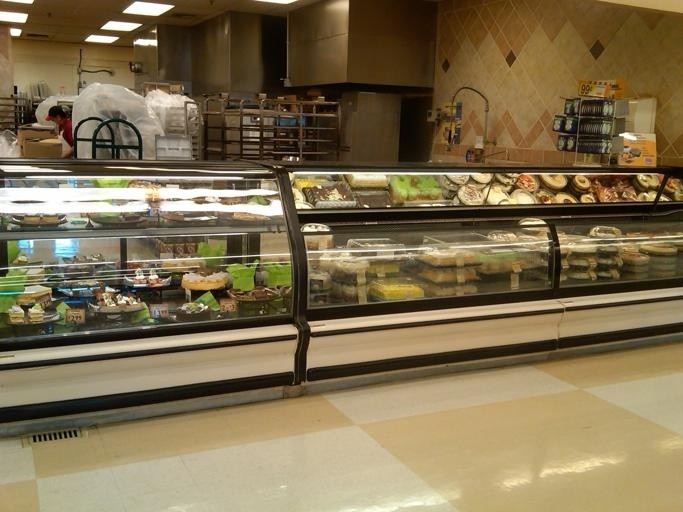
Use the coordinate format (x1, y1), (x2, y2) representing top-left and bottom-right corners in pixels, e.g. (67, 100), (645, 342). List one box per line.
(9, 306), (24, 318)
(16, 284), (50, 305)
(371, 282), (425, 302)
(124, 269), (174, 287)
(303, 180), (357, 208)
(389, 175), (442, 204)
(28, 304), (45, 322)
(419, 249), (481, 297)
(44, 213), (60, 222)
(176, 302), (210, 322)
(87, 289), (147, 313)
(23, 215), (41, 223)
(310, 272), (371, 305)
(181, 271), (228, 292)
(345, 173), (389, 189)
(351, 244), (404, 276)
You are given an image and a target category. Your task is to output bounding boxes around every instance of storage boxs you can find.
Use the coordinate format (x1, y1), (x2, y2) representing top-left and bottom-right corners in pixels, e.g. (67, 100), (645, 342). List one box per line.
(20, 139), (64, 160)
(16, 124), (55, 149)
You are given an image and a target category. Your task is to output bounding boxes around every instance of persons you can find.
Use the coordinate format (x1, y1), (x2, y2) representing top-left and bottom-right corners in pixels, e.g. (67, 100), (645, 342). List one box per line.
(44, 106), (75, 159)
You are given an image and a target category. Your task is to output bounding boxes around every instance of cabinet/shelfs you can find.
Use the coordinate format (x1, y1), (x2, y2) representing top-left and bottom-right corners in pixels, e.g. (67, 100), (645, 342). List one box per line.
(0, 158), (303, 424)
(273, 159), (683, 386)
(285, 0), (439, 89)
(333, 91), (404, 164)
(200, 91), (345, 160)
